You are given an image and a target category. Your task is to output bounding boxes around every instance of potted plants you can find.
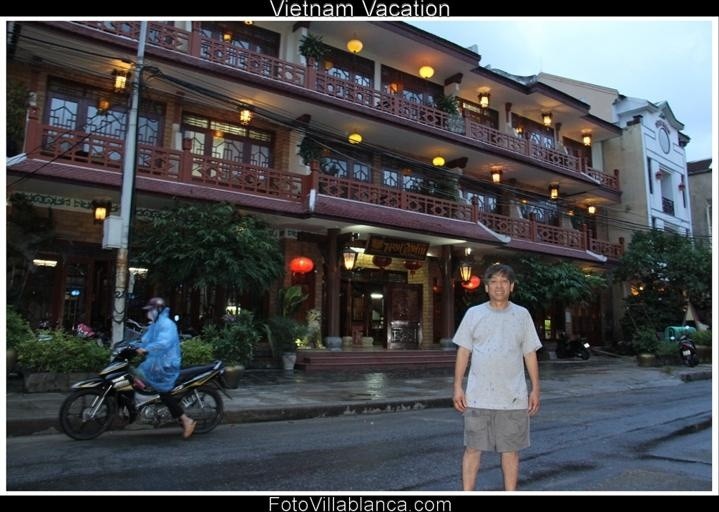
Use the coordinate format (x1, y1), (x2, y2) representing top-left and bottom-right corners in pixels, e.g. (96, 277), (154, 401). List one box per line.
(632, 325), (660, 368)
(267, 312), (311, 370)
(7, 303), (29, 374)
(201, 319), (264, 390)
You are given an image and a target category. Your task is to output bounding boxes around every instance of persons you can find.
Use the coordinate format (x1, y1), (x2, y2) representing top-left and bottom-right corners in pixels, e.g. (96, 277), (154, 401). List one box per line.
(451, 263), (544, 490)
(130, 296), (197, 440)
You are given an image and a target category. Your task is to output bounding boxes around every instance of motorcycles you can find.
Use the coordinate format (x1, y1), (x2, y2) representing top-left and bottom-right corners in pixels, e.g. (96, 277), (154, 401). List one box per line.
(59, 339), (231, 442)
(555, 329), (591, 360)
(678, 336), (699, 368)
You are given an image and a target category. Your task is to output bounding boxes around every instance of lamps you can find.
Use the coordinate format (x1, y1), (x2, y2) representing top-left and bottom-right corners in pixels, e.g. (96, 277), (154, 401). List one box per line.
(420, 66), (434, 79)
(542, 112), (553, 127)
(390, 80), (404, 93)
(90, 199), (112, 225)
(490, 169), (503, 182)
(342, 247), (357, 271)
(587, 204), (596, 215)
(582, 134), (592, 147)
(222, 31), (233, 43)
(548, 184), (560, 200)
(237, 105), (254, 125)
(114, 69), (128, 94)
(459, 259), (473, 284)
(347, 39), (364, 55)
(478, 94), (490, 109)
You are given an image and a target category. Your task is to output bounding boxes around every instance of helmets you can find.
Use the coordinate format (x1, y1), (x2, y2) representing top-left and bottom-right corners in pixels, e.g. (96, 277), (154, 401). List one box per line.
(143, 298), (166, 309)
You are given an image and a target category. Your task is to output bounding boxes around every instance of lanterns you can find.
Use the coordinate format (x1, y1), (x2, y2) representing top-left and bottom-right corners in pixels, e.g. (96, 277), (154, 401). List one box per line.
(289, 255), (314, 274)
(402, 260), (422, 274)
(347, 39), (363, 54)
(419, 65), (435, 79)
(372, 256), (391, 269)
(461, 275), (480, 292)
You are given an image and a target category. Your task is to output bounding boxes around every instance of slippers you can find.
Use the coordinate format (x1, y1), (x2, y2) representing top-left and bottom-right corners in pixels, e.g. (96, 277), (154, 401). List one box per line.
(184, 420), (197, 438)
